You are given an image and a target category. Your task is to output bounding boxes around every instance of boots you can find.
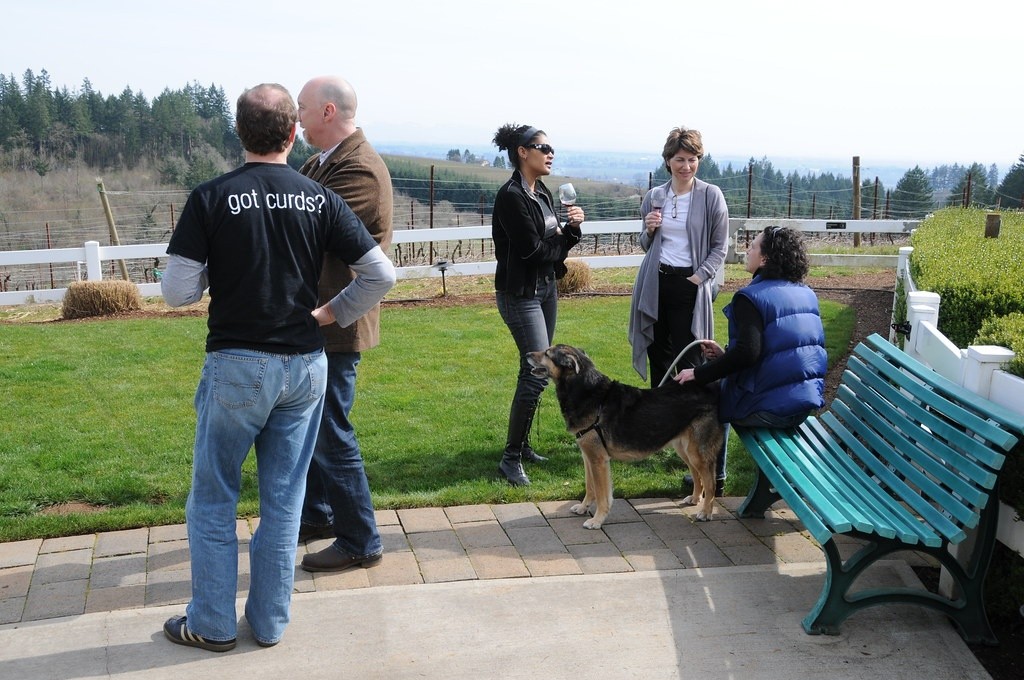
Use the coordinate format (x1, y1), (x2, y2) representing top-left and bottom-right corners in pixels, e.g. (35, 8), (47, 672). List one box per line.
(523, 405), (549, 462)
(498, 382), (541, 486)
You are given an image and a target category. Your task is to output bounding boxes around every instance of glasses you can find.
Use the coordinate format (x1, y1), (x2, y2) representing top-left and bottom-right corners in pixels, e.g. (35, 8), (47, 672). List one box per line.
(522, 143), (554, 154)
(772, 226), (786, 251)
(672, 195), (678, 219)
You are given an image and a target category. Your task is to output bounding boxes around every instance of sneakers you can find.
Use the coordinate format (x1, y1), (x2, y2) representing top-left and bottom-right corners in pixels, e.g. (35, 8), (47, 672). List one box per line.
(163, 615), (236, 651)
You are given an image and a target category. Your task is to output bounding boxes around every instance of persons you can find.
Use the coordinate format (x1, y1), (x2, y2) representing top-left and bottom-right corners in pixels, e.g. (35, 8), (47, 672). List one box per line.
(628, 126), (729, 390)
(297, 77), (392, 572)
(491, 124), (585, 485)
(673, 225), (827, 497)
(160, 84), (398, 652)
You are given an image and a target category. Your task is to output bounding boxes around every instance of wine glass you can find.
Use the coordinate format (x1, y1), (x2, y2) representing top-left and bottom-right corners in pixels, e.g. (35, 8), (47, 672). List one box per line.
(558, 182), (580, 225)
(651, 187), (666, 226)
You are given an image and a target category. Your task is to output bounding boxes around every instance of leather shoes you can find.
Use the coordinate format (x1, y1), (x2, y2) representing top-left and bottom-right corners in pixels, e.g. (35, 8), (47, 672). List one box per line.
(300, 544), (383, 572)
(299, 522), (335, 543)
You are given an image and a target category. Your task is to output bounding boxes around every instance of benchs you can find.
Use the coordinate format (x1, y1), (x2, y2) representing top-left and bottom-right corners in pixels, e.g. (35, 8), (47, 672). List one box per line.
(729, 332), (1024, 647)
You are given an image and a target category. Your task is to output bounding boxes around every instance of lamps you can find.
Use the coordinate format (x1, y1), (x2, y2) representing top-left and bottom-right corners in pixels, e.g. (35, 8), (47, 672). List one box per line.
(430, 261), (454, 298)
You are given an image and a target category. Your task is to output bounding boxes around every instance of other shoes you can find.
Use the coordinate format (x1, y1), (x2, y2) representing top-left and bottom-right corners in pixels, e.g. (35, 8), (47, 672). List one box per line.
(683, 475), (725, 498)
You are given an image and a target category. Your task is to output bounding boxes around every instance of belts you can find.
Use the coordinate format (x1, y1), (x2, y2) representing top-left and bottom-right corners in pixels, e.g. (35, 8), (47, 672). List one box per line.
(658, 261), (693, 276)
(538, 271), (556, 286)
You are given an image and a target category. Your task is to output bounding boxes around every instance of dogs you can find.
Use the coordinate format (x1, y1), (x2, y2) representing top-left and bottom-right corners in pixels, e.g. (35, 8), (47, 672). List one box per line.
(525, 342), (728, 531)
